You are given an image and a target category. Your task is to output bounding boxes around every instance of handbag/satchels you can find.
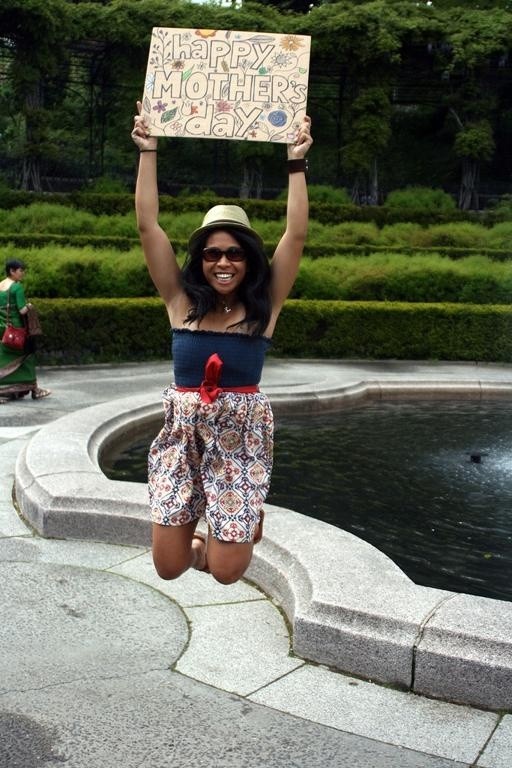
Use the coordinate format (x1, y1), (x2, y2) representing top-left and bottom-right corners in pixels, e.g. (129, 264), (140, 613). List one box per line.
(2, 325), (26, 354)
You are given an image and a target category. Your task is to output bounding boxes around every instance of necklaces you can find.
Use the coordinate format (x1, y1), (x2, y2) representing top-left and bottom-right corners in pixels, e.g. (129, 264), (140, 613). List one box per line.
(218, 297), (237, 315)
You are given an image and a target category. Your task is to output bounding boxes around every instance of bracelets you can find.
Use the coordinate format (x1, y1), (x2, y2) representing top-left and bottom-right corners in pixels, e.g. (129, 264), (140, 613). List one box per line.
(288, 158), (309, 173)
(138, 148), (159, 154)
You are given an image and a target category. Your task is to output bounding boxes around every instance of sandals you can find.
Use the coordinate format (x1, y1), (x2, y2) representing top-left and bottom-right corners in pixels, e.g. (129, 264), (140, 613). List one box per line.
(30, 388), (52, 400)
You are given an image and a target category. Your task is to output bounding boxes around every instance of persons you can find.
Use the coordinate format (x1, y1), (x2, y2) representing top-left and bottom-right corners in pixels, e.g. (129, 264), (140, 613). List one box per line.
(130, 96), (316, 587)
(0, 256), (53, 407)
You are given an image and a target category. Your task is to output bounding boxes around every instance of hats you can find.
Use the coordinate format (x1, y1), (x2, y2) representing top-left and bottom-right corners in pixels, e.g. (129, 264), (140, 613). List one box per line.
(187, 203), (265, 252)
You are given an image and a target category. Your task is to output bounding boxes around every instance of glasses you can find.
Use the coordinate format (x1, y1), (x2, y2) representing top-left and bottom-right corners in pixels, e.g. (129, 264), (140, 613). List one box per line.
(203, 246), (247, 264)
